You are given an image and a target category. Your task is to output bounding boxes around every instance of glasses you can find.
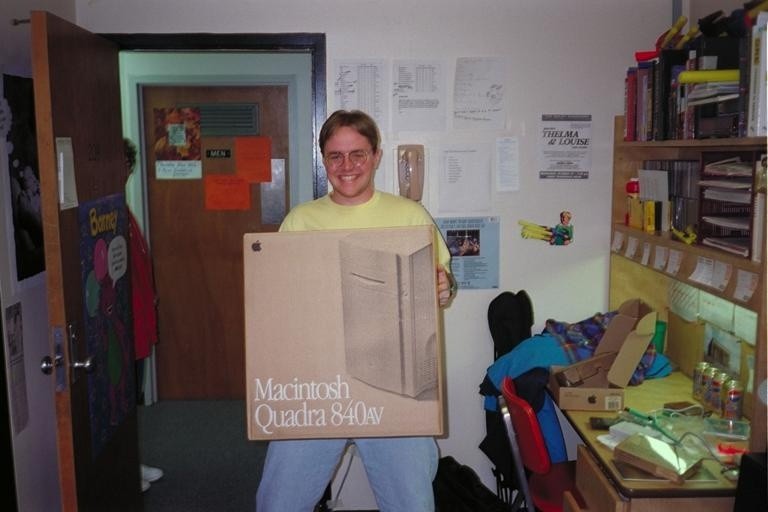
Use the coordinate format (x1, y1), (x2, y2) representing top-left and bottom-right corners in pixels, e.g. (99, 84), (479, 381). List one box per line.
(322, 146), (375, 168)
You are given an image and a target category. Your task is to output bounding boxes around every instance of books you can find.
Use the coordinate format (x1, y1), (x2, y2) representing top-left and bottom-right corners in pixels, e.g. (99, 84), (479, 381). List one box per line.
(621, 26), (762, 263)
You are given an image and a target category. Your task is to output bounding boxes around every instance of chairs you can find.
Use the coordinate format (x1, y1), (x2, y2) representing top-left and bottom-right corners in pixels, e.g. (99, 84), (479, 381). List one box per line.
(493, 377), (562, 512)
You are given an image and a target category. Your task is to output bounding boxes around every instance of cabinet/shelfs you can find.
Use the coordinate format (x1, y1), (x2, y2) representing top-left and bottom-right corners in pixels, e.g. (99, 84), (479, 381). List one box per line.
(607, 105), (768, 295)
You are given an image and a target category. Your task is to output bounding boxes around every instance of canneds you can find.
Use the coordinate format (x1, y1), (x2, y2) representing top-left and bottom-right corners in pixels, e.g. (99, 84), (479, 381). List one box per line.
(693, 362), (743, 422)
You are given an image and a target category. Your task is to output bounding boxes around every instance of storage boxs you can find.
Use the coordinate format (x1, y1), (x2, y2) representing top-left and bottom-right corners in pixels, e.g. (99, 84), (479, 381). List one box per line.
(243, 224), (448, 438)
(549, 297), (657, 413)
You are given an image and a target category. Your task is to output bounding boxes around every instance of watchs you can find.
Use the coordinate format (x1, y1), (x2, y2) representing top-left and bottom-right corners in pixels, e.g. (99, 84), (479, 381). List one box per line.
(447, 273), (456, 298)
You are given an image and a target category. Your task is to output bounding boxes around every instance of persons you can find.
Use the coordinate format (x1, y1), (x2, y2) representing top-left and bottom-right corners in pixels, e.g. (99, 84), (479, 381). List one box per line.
(254, 111), (458, 512)
(119, 136), (164, 494)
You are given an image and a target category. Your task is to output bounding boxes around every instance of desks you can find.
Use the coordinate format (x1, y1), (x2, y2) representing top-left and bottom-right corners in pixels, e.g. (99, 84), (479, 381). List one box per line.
(557, 368), (768, 511)
